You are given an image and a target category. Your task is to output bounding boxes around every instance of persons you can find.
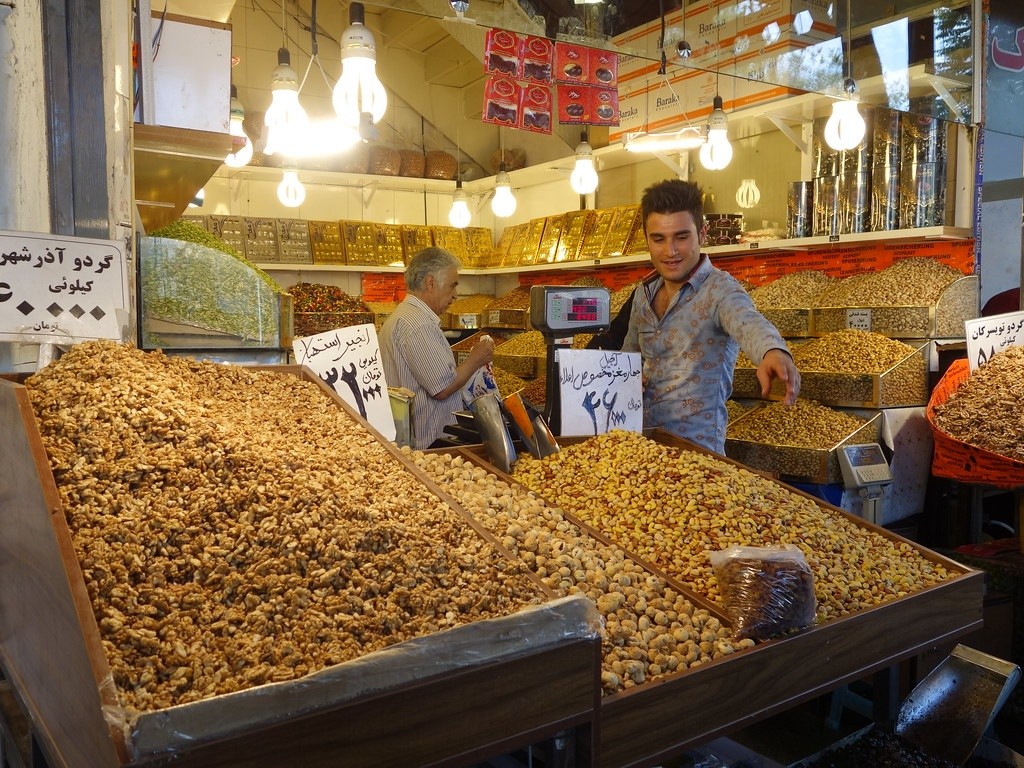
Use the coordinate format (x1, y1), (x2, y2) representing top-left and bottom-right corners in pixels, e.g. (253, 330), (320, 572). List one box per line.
(377, 247), (496, 450)
(583, 180), (801, 459)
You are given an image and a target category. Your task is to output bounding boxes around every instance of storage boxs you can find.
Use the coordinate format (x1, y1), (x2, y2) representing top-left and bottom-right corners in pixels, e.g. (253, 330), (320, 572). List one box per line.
(607, 0), (838, 146)
(179, 201), (655, 266)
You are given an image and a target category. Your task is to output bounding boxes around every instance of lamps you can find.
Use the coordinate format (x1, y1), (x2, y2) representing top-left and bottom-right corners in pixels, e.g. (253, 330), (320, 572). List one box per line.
(450, 0), (469, 13)
(735, 139), (761, 209)
(677, 0), (690, 60)
(621, 67), (703, 153)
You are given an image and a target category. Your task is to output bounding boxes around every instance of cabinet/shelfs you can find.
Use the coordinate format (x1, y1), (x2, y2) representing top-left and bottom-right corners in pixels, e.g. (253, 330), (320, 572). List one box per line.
(186, 63), (974, 277)
(131, 6), (233, 233)
